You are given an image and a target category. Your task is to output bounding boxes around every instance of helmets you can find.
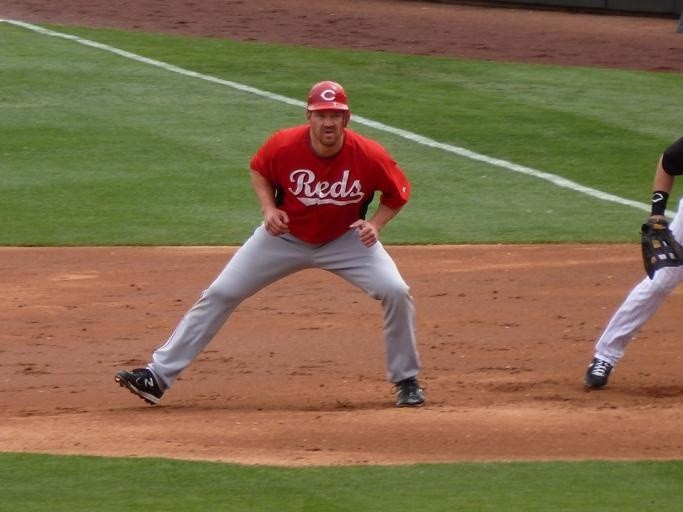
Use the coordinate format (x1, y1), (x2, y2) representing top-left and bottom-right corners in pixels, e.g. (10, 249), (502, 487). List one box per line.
(308, 81), (350, 127)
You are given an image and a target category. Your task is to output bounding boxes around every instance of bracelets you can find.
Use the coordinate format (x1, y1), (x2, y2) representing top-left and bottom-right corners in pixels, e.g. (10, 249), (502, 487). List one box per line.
(649, 190), (668, 218)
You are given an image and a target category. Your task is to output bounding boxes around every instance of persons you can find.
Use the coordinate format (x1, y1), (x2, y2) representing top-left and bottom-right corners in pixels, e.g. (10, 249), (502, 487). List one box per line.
(581, 134), (682, 389)
(113, 80), (429, 408)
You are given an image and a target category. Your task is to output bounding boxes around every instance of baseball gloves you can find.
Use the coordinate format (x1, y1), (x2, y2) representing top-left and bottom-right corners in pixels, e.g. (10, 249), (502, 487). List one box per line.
(640, 217), (681, 278)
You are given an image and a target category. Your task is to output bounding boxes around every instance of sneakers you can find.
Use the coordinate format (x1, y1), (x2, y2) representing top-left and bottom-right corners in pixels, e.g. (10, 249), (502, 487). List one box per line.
(395, 375), (424, 406)
(585, 358), (613, 386)
(115, 368), (164, 405)
